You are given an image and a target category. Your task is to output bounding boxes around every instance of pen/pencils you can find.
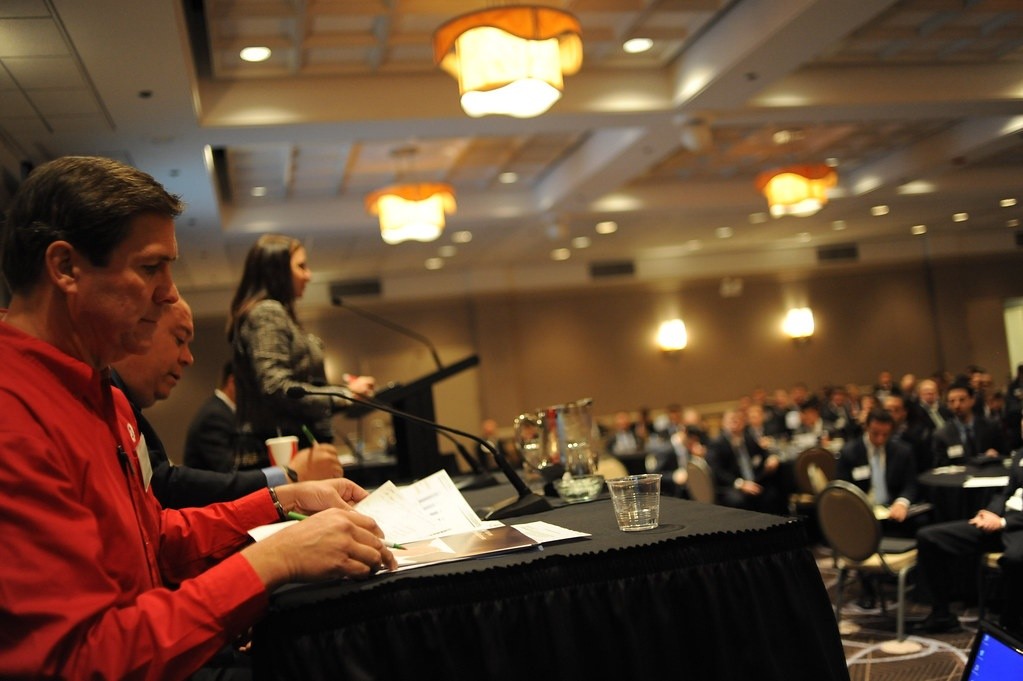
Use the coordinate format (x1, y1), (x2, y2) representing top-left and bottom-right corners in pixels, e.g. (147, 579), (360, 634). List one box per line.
(116, 445), (136, 478)
(302, 425), (318, 445)
(287, 512), (409, 551)
(341, 373), (374, 390)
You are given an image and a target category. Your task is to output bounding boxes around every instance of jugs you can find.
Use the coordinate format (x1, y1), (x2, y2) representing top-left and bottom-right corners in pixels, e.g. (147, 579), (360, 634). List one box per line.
(513, 398), (604, 503)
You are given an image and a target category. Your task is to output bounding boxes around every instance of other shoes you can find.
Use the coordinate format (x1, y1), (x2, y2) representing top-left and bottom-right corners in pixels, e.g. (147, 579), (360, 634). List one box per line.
(848, 599), (882, 614)
(905, 613), (962, 634)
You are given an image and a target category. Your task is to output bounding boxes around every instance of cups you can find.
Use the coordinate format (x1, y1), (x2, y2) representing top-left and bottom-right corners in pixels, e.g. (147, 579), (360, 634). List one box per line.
(265, 436), (299, 466)
(604, 474), (662, 532)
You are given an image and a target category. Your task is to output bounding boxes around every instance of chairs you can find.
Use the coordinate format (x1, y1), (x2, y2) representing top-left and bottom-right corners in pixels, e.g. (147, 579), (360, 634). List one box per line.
(793, 447), (1004, 644)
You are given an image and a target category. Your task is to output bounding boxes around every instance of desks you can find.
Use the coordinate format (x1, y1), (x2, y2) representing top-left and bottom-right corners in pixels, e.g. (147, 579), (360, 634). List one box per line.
(236, 464), (852, 681)
(920, 464), (1011, 488)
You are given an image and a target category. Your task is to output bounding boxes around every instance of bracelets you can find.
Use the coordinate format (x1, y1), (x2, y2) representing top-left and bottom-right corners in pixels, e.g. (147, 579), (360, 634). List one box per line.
(282, 465), (298, 482)
(269, 487), (287, 522)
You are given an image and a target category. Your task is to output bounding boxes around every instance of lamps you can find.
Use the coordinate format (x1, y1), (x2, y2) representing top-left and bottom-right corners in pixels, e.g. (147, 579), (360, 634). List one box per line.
(365, 181), (456, 245)
(753, 161), (839, 219)
(433, 4), (584, 120)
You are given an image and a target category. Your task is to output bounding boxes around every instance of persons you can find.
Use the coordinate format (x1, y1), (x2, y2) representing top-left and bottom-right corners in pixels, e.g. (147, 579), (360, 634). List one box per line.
(226, 232), (376, 452)
(108, 292), (342, 510)
(904, 363), (1023, 634)
(477, 419), (509, 470)
(596, 371), (937, 539)
(0, 156), (398, 681)
(183, 360), (272, 472)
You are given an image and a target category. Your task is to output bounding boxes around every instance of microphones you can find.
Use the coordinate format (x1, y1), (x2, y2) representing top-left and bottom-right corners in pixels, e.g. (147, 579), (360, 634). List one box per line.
(330, 296), (443, 370)
(287, 379), (553, 520)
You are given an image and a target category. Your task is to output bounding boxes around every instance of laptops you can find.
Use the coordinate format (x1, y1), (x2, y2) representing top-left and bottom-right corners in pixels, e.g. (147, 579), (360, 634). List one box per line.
(959, 621), (1023, 681)
